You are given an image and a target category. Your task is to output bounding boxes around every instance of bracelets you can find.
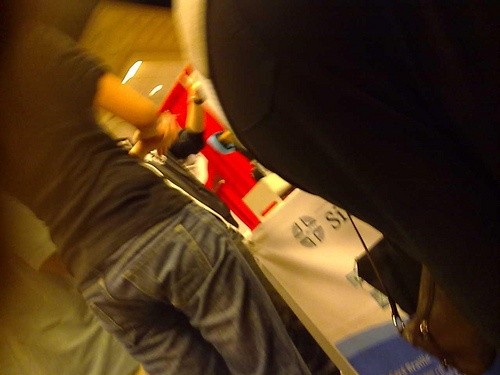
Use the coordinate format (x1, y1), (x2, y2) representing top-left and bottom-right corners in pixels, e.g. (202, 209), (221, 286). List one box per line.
(193, 96), (205, 105)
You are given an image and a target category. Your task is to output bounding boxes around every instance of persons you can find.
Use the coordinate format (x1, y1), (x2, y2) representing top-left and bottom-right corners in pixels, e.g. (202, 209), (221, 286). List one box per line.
(1, 1), (313, 375)
(0, 189), (148, 375)
(207, 2), (500, 352)
(144, 70), (240, 230)
